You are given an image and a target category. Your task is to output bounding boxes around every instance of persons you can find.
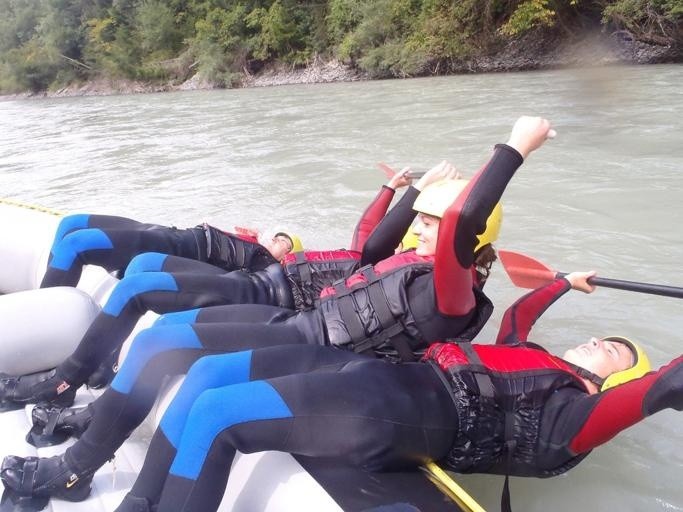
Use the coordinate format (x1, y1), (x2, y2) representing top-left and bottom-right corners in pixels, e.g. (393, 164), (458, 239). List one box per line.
(0, 114), (558, 503)
(0, 159), (464, 410)
(37, 213), (304, 288)
(111, 269), (682, 512)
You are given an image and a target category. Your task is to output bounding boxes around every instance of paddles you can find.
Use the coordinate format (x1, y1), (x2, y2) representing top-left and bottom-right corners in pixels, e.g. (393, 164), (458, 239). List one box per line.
(376, 162), (428, 181)
(234, 226), (257, 239)
(497, 249), (683, 300)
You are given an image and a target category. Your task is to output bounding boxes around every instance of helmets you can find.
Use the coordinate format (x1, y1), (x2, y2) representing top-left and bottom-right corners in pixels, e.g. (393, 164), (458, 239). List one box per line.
(411, 178), (502, 254)
(599, 336), (652, 391)
(275, 231), (304, 254)
(401, 224), (421, 252)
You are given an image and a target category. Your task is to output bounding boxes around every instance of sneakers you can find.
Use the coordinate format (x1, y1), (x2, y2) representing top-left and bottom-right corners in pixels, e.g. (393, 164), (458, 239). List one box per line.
(1, 453), (92, 502)
(33, 401), (96, 441)
(88, 346), (120, 390)
(1, 372), (75, 409)
